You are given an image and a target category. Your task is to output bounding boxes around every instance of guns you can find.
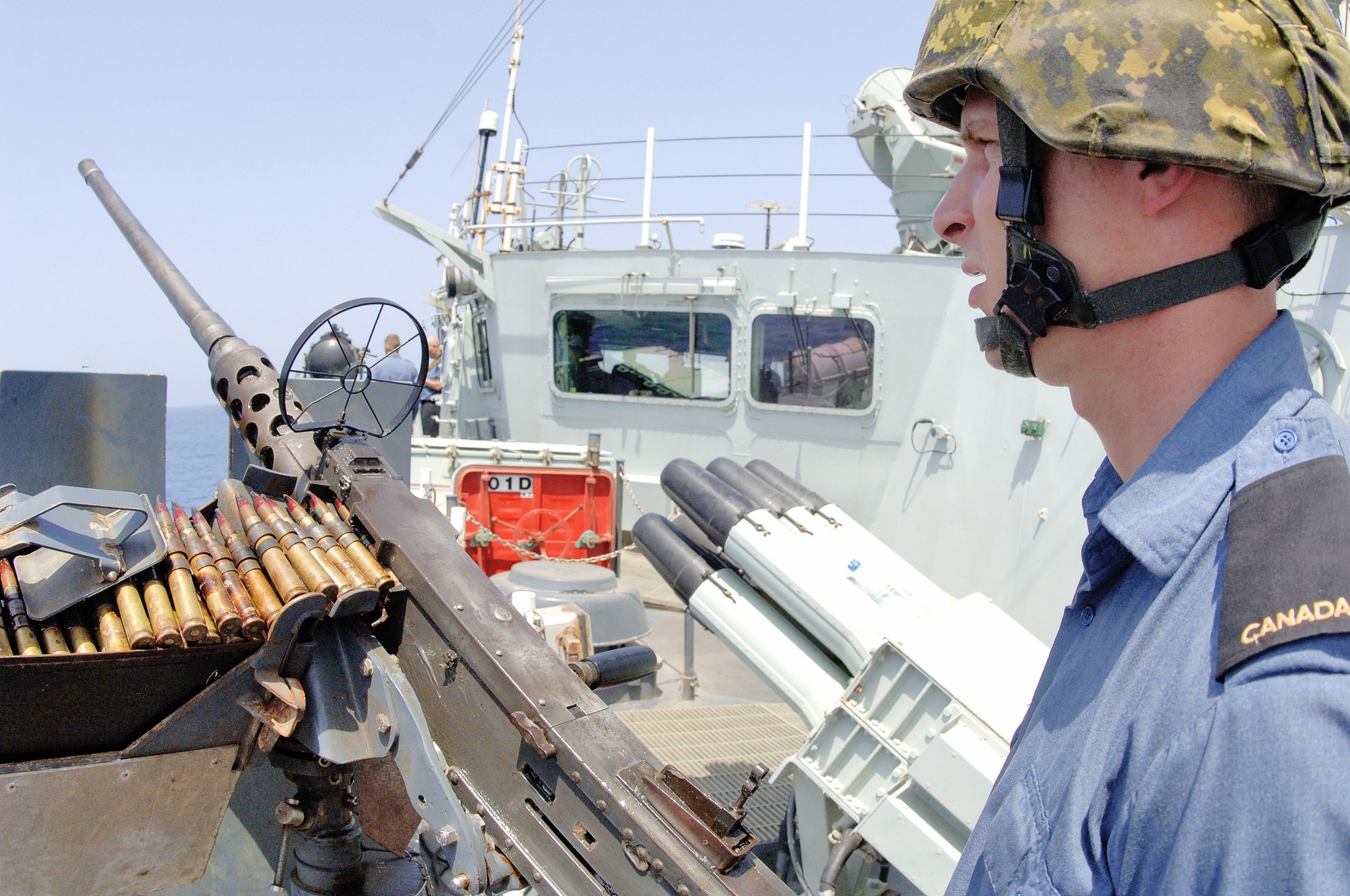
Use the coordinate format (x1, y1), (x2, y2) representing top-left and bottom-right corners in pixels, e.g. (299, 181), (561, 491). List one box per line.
(74, 144), (798, 895)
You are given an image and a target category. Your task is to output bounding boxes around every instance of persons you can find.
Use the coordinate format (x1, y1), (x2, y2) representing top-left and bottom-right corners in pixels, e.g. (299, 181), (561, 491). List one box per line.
(904, 0), (1350, 896)
(371, 334), (420, 435)
(758, 355), (782, 404)
(419, 337), (441, 437)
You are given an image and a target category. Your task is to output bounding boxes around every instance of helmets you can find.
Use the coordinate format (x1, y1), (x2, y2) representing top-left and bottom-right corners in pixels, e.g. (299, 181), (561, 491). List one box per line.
(902, 0), (1350, 199)
